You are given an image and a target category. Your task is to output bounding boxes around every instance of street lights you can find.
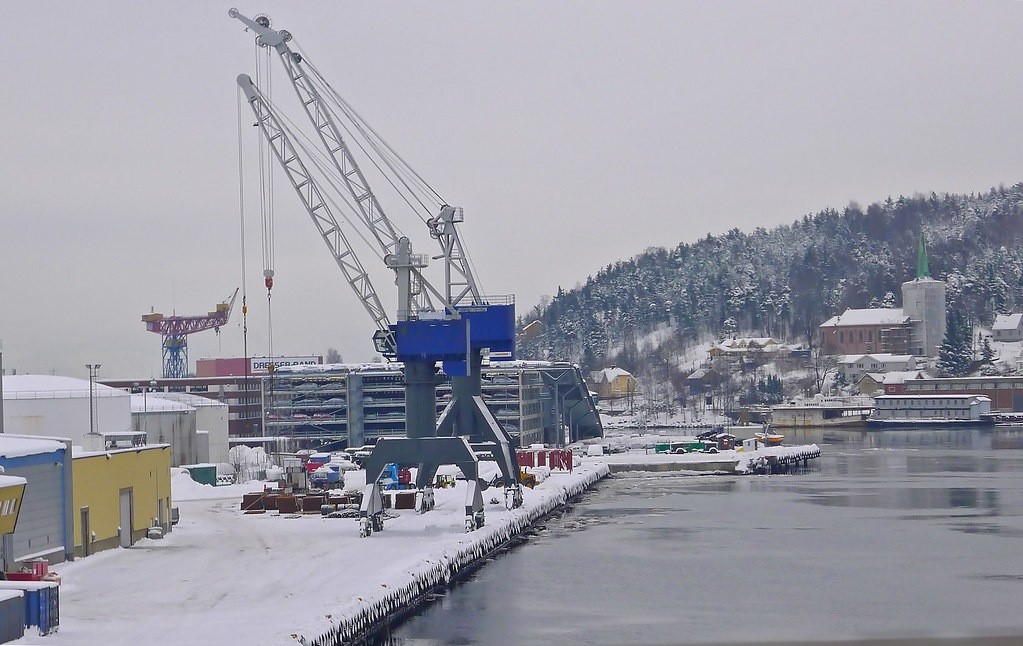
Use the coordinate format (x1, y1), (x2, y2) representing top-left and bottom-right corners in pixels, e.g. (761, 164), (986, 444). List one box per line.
(85, 364), (102, 433)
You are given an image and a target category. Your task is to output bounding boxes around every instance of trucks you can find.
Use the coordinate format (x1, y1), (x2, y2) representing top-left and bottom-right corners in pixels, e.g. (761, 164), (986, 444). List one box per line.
(305, 452), (346, 490)
(377, 463), (458, 490)
(478, 460), (539, 490)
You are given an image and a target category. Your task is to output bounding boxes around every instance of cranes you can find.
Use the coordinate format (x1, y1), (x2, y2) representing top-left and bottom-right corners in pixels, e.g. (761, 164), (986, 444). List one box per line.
(227, 6), (518, 378)
(140, 287), (241, 378)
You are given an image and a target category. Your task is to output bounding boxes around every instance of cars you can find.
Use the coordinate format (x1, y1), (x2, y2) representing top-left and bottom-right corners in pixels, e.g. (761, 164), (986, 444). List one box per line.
(263, 382), (345, 421)
(440, 374), (519, 434)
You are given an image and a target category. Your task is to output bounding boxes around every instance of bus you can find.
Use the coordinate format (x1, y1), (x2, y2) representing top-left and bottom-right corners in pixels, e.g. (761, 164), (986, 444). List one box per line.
(655, 440), (720, 454)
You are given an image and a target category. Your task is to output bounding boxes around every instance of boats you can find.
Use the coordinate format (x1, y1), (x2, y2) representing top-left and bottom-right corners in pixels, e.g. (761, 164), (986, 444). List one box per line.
(724, 387), (874, 426)
(753, 422), (785, 446)
